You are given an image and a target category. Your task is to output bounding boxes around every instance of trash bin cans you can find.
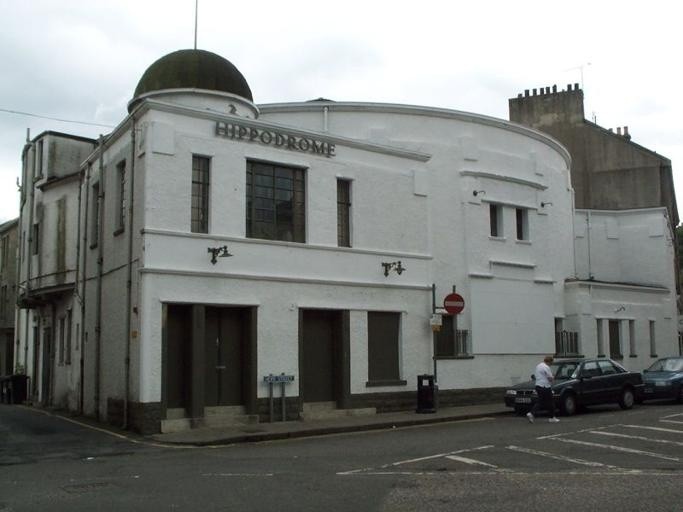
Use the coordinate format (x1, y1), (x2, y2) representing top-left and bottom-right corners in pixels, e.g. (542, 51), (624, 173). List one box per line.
(416, 374), (438, 413)
(0, 374), (30, 405)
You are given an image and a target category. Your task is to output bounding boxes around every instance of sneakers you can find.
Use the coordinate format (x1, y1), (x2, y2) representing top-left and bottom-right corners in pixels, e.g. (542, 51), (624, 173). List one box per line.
(549, 416), (559, 423)
(527, 412), (535, 423)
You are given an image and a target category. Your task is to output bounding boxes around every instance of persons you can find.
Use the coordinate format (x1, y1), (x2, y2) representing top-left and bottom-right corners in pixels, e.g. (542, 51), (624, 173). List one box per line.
(526, 357), (560, 424)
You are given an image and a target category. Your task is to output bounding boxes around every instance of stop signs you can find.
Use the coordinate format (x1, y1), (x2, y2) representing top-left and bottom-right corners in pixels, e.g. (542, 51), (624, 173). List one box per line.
(443, 293), (464, 315)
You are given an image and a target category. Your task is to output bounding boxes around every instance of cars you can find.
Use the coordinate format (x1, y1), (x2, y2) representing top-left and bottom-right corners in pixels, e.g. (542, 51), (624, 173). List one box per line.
(634, 356), (682, 405)
(503, 358), (645, 417)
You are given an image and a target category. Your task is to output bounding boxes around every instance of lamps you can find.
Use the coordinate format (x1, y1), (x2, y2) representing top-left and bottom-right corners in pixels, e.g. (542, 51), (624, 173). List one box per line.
(208, 245), (233, 264)
(382, 261), (405, 277)
